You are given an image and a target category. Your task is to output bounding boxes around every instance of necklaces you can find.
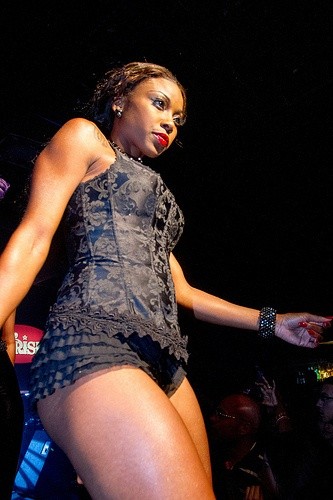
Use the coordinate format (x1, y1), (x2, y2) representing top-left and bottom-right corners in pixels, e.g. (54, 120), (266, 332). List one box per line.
(109, 137), (142, 163)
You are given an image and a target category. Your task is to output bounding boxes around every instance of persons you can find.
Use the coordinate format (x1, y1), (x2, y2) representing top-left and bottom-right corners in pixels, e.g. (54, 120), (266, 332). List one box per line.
(0, 60), (333, 500)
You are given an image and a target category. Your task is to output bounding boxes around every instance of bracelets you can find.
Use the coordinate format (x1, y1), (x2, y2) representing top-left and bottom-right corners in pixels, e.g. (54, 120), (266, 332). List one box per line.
(259, 308), (275, 338)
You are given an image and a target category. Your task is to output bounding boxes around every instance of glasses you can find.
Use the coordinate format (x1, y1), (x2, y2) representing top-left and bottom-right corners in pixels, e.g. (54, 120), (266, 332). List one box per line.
(216, 410), (250, 424)
(320, 392), (333, 401)
